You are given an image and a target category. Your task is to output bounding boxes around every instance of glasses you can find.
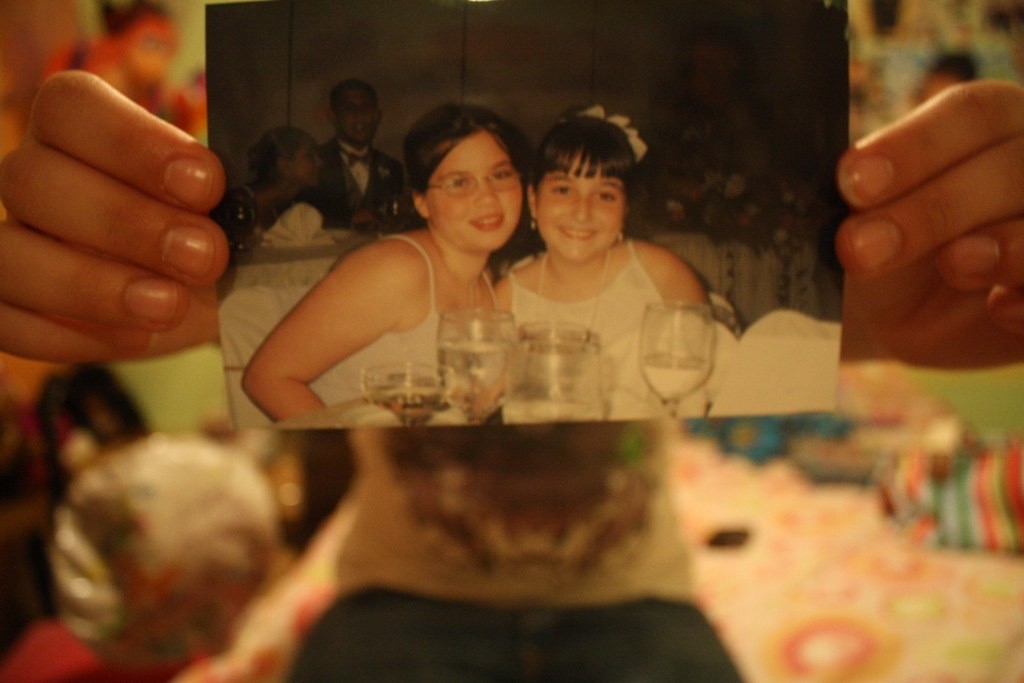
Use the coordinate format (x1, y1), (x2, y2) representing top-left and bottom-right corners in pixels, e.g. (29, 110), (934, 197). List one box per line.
(425, 167), (522, 198)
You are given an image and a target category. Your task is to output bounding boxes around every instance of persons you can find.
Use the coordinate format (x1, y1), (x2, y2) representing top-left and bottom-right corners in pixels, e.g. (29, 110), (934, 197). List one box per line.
(296, 79), (405, 231)
(222, 126), (324, 250)
(0, 0), (1024, 683)
(492, 119), (711, 418)
(241, 103), (527, 427)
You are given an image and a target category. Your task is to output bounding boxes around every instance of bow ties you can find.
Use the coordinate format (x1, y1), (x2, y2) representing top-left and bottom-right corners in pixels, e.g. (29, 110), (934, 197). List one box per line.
(341, 147), (369, 167)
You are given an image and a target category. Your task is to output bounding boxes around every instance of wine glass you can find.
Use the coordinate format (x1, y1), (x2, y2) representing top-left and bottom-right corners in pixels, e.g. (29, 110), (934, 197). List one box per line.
(436, 307), (520, 425)
(637, 299), (716, 417)
(359, 362), (456, 426)
(226, 205), (255, 254)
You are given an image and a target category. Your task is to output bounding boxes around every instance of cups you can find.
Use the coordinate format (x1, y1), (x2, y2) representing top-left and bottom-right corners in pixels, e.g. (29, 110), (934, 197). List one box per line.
(502, 319), (606, 421)
(378, 192), (409, 233)
